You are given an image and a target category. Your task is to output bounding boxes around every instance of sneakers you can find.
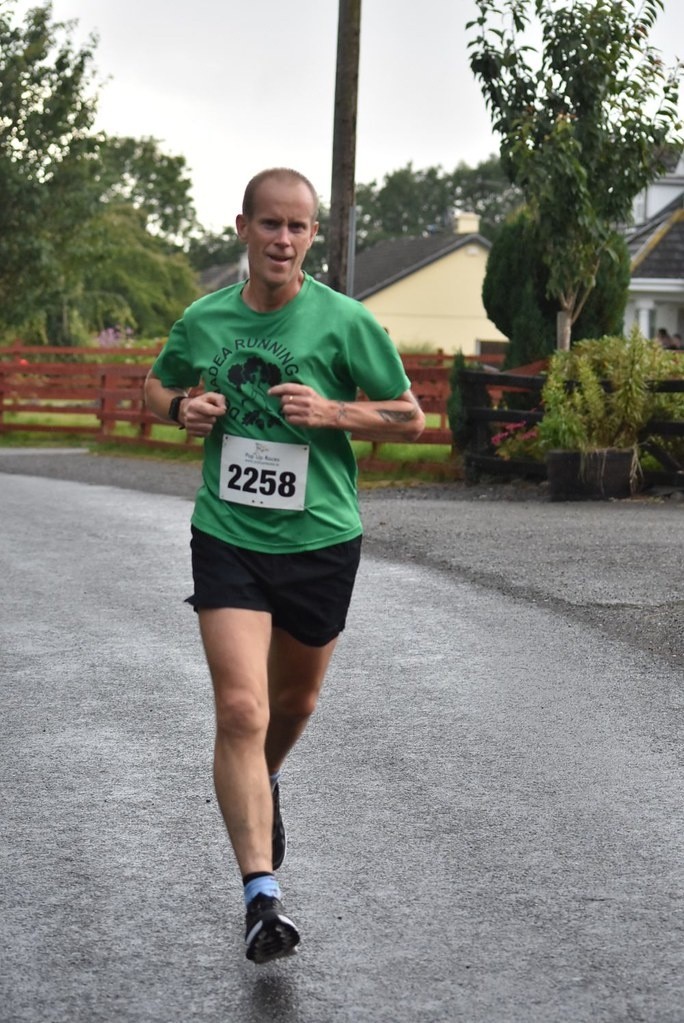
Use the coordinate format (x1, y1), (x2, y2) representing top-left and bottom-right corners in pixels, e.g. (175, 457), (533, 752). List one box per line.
(244, 892), (300, 966)
(273, 783), (286, 872)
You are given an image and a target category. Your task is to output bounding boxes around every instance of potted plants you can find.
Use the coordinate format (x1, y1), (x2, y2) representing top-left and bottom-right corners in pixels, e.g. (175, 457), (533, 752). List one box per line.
(543, 327), (643, 501)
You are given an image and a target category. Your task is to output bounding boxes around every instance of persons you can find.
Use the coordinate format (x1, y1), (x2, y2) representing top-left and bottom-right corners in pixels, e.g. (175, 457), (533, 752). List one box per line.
(656, 327), (684, 349)
(144, 168), (424, 963)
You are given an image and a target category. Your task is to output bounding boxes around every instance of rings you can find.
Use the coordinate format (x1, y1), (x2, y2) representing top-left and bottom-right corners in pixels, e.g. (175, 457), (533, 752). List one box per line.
(288, 394), (295, 405)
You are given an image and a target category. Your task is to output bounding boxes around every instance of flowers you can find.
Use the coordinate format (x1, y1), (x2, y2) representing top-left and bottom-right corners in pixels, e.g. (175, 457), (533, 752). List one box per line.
(490, 421), (542, 459)
(91, 326), (159, 363)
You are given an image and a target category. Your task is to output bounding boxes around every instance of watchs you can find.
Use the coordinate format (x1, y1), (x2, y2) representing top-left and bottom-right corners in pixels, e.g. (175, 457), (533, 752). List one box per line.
(167, 396), (186, 431)
